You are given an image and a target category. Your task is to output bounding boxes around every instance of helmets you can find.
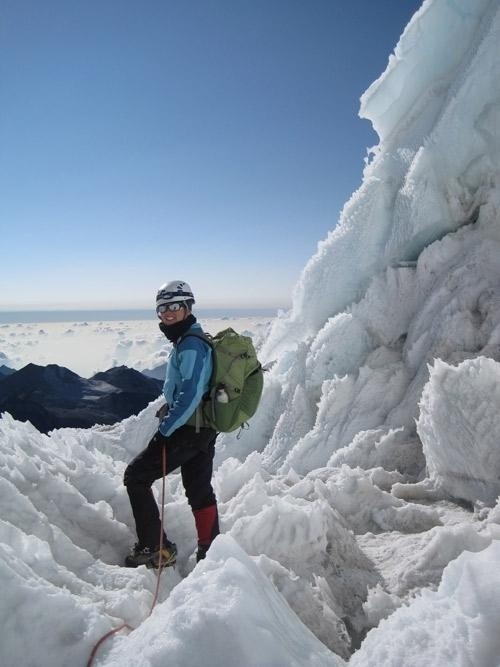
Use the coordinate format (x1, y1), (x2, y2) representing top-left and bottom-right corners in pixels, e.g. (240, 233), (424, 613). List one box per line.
(155, 280), (195, 311)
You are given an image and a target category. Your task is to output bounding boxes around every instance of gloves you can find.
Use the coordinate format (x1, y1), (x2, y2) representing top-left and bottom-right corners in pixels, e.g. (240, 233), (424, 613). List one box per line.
(148, 432), (171, 460)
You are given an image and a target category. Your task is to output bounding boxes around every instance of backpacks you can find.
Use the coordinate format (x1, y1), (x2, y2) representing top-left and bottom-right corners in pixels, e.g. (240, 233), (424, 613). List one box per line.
(177, 327), (263, 440)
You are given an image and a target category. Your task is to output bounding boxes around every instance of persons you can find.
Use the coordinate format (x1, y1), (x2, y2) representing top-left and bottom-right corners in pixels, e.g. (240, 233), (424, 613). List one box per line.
(119, 278), (222, 571)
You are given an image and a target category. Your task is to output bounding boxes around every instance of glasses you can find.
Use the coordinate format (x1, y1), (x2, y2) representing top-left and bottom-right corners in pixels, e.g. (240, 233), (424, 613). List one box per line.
(157, 303), (185, 313)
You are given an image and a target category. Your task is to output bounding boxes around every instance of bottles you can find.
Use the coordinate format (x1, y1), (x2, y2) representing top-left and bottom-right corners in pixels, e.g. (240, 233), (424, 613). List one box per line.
(216, 389), (230, 404)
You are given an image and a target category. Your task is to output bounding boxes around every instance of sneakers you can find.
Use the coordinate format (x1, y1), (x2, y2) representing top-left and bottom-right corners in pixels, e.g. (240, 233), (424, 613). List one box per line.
(124, 540), (178, 568)
